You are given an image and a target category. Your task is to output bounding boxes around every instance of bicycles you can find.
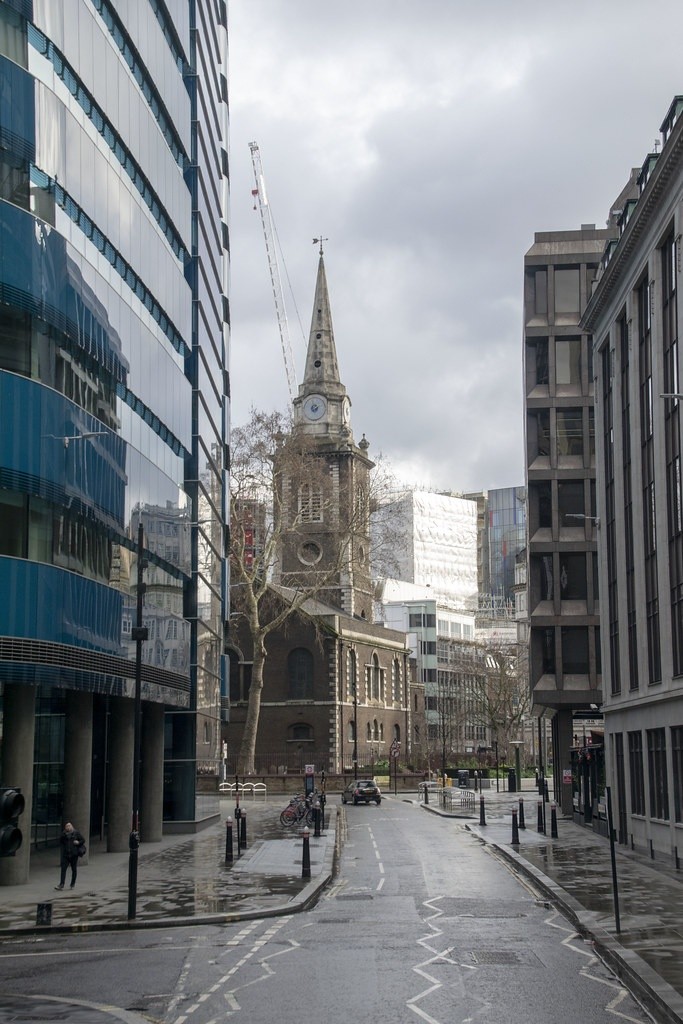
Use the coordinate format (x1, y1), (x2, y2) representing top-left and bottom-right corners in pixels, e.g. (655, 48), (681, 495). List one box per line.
(280, 788), (322, 827)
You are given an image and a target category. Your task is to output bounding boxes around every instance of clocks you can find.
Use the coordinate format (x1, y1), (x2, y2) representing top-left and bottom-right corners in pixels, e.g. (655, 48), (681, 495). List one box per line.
(304, 398), (326, 421)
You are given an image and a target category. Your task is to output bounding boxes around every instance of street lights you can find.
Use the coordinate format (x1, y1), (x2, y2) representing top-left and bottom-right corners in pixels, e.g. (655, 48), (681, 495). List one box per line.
(509, 741), (524, 790)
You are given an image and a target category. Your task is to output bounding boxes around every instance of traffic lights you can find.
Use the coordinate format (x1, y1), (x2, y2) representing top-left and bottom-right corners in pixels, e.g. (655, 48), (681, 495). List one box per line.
(0, 788), (25, 857)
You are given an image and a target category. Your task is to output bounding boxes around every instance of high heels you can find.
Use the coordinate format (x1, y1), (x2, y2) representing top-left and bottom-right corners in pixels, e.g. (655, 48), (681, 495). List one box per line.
(69, 883), (76, 890)
(54, 883), (64, 891)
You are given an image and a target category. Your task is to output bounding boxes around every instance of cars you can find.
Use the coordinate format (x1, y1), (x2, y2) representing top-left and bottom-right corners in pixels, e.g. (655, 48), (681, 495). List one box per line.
(342, 780), (381, 806)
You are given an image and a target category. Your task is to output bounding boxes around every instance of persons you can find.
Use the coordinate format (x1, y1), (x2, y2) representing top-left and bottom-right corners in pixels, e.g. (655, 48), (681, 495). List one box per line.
(53, 821), (85, 890)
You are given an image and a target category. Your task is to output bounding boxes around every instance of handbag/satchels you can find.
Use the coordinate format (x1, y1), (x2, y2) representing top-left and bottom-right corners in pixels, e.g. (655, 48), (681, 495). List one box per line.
(72, 831), (87, 858)
